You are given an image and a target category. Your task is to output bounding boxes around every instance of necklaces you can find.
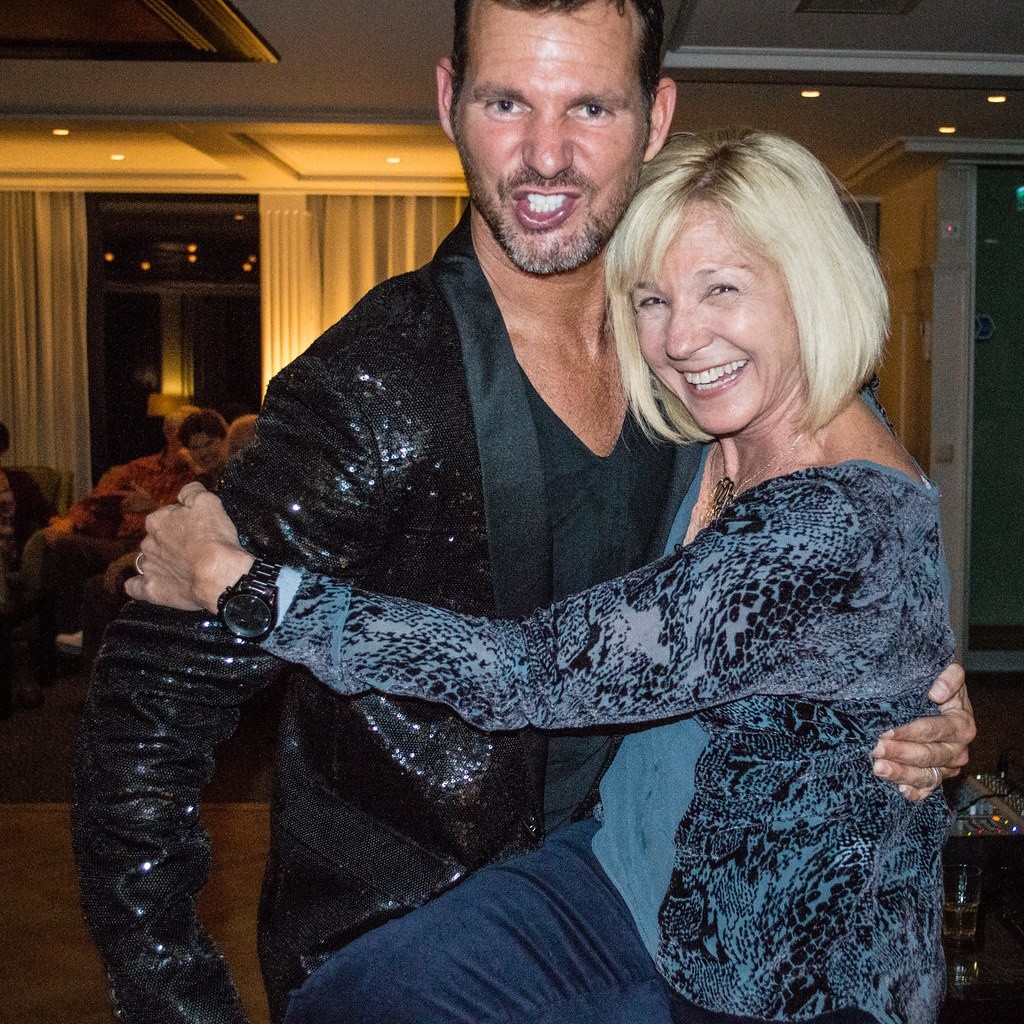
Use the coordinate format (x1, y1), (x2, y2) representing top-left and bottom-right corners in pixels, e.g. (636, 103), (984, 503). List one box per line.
(701, 423), (819, 524)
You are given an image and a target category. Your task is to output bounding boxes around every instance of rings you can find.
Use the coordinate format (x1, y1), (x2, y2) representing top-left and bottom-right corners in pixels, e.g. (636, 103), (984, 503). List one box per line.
(134, 552), (144, 575)
(931, 767), (941, 786)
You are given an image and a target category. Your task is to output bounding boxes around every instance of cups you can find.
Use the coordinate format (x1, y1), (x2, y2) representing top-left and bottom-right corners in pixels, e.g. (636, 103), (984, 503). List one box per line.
(943, 865), (980, 942)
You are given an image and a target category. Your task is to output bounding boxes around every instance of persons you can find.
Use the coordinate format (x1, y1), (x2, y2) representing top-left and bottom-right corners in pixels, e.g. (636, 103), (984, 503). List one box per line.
(122, 134), (959, 1024)
(0, 401), (262, 714)
(70, 0), (979, 1024)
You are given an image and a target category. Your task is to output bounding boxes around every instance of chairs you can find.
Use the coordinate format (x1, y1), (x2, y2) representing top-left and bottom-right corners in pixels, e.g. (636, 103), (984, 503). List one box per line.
(3, 466), (74, 572)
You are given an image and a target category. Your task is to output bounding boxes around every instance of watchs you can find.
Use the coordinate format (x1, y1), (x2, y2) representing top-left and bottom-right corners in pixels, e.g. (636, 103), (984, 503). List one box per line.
(216, 543), (279, 664)
